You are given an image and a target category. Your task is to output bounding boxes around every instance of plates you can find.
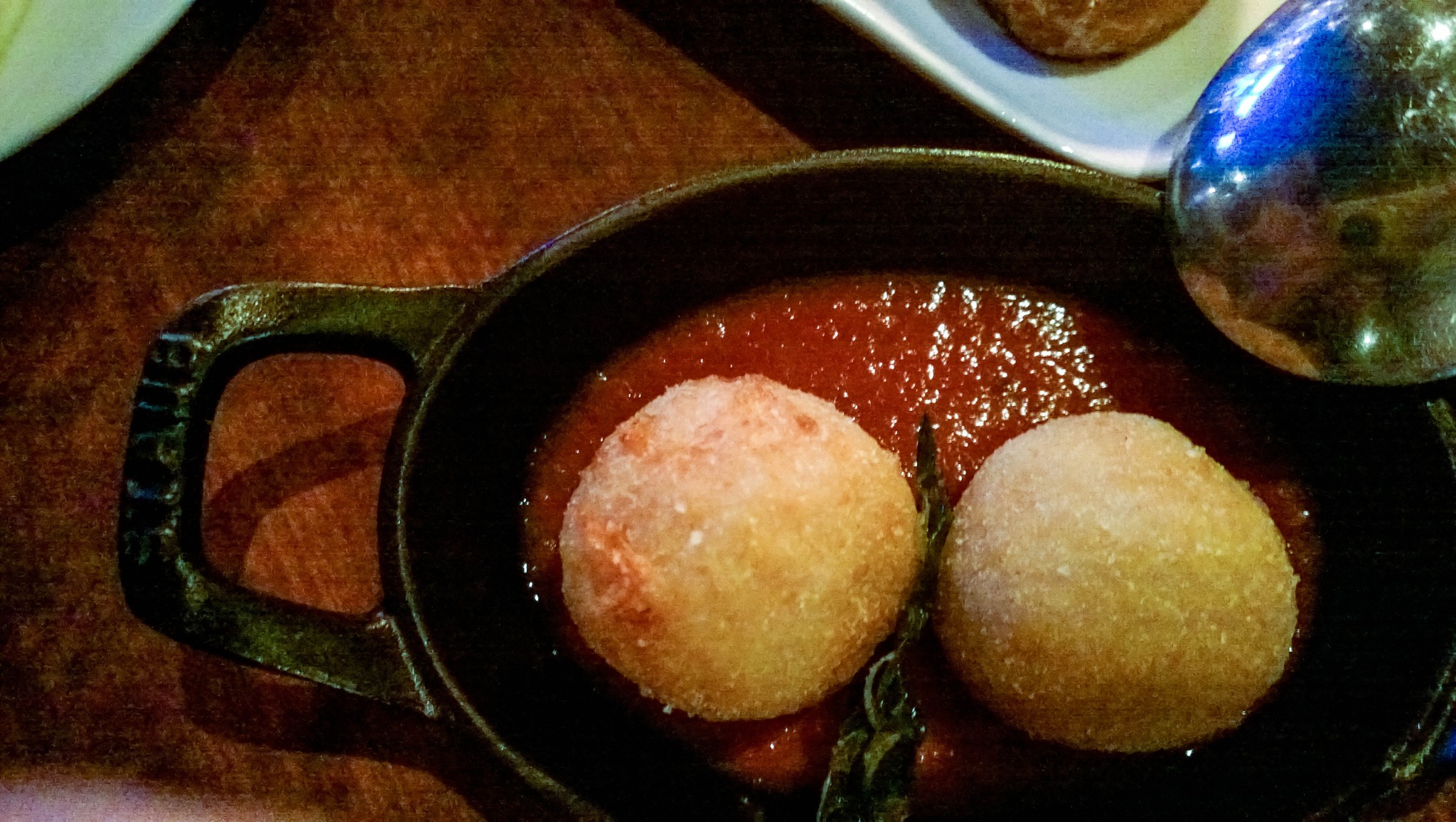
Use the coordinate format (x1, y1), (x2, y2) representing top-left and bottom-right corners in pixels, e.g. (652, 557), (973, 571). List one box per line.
(808, 1), (1287, 186)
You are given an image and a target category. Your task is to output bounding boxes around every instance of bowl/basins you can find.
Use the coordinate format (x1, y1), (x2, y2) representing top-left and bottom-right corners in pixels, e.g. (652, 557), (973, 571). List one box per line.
(112, 147), (1455, 821)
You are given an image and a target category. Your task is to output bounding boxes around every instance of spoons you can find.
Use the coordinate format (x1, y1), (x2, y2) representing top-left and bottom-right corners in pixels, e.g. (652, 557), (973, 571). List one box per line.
(1162, 0), (1454, 387)
